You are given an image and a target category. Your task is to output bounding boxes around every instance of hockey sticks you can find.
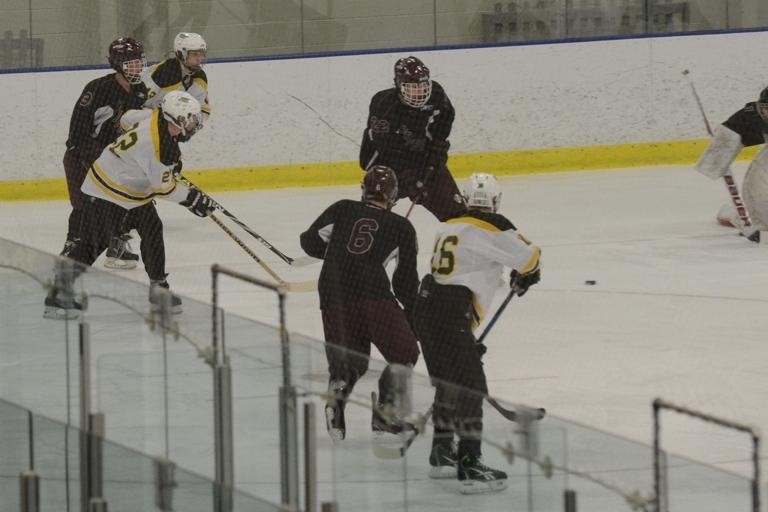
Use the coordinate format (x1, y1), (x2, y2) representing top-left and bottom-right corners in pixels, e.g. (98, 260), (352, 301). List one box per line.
(208, 210), (319, 292)
(678, 67), (760, 244)
(175, 174), (326, 267)
(486, 393), (545, 422)
(371, 288), (513, 458)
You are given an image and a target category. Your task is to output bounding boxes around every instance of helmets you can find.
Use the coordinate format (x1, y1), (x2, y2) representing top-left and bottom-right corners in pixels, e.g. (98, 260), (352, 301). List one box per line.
(174, 32), (207, 69)
(362, 165), (398, 210)
(395, 57), (432, 108)
(161, 90), (203, 136)
(109, 37), (147, 85)
(463, 173), (501, 213)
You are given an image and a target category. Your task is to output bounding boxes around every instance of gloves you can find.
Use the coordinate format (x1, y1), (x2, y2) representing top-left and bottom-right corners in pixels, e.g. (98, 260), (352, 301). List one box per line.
(510, 259), (540, 296)
(179, 188), (216, 217)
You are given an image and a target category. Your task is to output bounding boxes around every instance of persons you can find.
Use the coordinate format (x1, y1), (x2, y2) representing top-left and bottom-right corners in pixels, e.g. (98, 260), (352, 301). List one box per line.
(409, 171), (544, 485)
(40, 37), (153, 294)
(693, 84), (768, 235)
(145, 30), (212, 126)
(297, 166), (421, 442)
(43, 91), (224, 314)
(357, 53), (469, 223)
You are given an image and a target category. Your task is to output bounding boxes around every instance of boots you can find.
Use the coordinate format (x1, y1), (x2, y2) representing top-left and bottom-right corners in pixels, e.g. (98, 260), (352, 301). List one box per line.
(325, 364), (359, 441)
(106, 233), (139, 261)
(149, 273), (181, 305)
(372, 390), (416, 431)
(45, 268), (84, 310)
(430, 404), (507, 479)
